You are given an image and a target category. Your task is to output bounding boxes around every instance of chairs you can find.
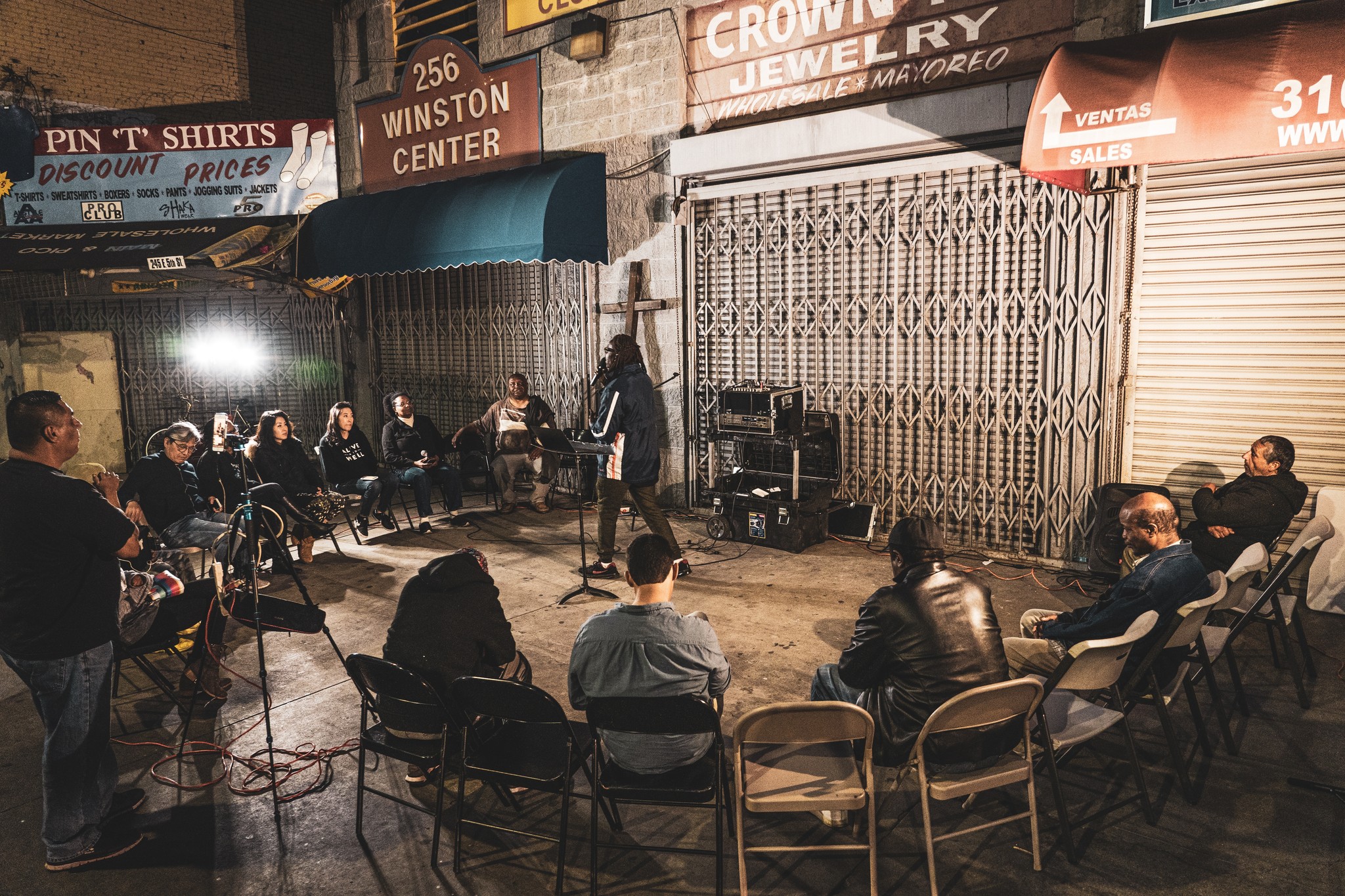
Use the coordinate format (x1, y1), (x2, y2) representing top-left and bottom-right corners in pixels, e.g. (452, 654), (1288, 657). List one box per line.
(577, 692), (735, 896)
(446, 674), (618, 896)
(1031, 514), (1335, 867)
(341, 652), (510, 870)
(879, 679), (1046, 896)
(108, 429), (571, 715)
(733, 700), (879, 896)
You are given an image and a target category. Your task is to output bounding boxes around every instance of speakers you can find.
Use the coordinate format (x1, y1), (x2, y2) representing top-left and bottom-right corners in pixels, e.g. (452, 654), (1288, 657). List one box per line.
(1088, 484), (1170, 577)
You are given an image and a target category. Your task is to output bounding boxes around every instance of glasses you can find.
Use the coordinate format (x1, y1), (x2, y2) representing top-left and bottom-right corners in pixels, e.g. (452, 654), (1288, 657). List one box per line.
(228, 424), (240, 434)
(394, 399), (413, 408)
(172, 439), (197, 454)
(603, 347), (613, 353)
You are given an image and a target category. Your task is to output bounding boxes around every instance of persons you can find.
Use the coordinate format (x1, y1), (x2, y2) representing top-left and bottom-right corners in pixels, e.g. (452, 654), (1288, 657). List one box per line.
(1002, 492), (1212, 756)
(1119, 436), (1308, 586)
(382, 391), (470, 534)
(809, 515), (1024, 826)
(578, 333), (692, 580)
(0, 391), (147, 870)
(118, 422), (270, 590)
(451, 373), (558, 513)
(65, 463), (232, 699)
(567, 533), (731, 779)
(197, 416), (336, 576)
(375, 547), (532, 782)
(253, 409), (345, 563)
(320, 401), (397, 538)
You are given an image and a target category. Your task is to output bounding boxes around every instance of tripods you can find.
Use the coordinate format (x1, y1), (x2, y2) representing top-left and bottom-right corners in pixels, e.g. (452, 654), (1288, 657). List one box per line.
(531, 426), (621, 609)
(178, 443), (379, 815)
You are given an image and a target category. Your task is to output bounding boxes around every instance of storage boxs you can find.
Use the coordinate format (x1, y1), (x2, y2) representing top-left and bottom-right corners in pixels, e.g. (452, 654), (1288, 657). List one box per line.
(706, 411), (842, 554)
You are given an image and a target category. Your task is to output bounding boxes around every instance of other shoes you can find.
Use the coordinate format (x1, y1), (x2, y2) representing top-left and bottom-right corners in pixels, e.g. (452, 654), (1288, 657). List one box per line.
(298, 529), (315, 563)
(272, 556), (302, 574)
(404, 763), (440, 784)
(499, 502), (515, 514)
(176, 620), (201, 634)
(508, 783), (529, 794)
(233, 571), (270, 589)
(291, 523), (303, 546)
(153, 637), (194, 654)
(449, 514), (469, 527)
(419, 521), (433, 534)
(531, 502), (549, 512)
(811, 808), (849, 828)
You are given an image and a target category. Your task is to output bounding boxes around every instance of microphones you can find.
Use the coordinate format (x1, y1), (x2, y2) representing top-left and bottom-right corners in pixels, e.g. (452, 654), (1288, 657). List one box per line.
(421, 450), (432, 458)
(589, 357), (606, 386)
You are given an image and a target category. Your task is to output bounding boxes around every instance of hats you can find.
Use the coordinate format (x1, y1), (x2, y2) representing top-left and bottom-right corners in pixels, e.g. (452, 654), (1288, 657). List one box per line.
(888, 516), (944, 550)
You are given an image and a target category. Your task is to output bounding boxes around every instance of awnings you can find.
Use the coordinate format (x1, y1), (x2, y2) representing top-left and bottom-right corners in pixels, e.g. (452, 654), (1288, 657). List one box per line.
(1018, 16), (1345, 193)
(0, 213), (309, 273)
(291, 152), (609, 300)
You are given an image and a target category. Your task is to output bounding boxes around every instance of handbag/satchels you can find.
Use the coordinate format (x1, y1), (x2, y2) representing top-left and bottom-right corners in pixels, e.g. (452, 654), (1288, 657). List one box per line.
(148, 569), (185, 602)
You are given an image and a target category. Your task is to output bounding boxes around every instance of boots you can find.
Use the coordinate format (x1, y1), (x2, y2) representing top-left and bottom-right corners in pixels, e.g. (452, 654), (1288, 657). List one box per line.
(184, 643), (234, 700)
(184, 655), (232, 688)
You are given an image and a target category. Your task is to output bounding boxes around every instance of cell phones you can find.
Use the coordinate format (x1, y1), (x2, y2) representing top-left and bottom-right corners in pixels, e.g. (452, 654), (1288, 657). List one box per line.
(360, 476), (378, 480)
(207, 498), (222, 512)
(212, 413), (228, 451)
(425, 459), (436, 466)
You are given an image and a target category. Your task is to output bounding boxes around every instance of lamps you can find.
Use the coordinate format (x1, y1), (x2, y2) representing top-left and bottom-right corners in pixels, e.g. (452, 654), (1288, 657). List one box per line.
(567, 14), (608, 65)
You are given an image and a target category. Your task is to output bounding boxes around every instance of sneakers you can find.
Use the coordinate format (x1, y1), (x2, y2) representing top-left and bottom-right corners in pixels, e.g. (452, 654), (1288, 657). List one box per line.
(353, 513), (369, 538)
(372, 508), (396, 532)
(45, 825), (143, 869)
(104, 787), (147, 817)
(677, 558), (692, 578)
(578, 560), (620, 579)
